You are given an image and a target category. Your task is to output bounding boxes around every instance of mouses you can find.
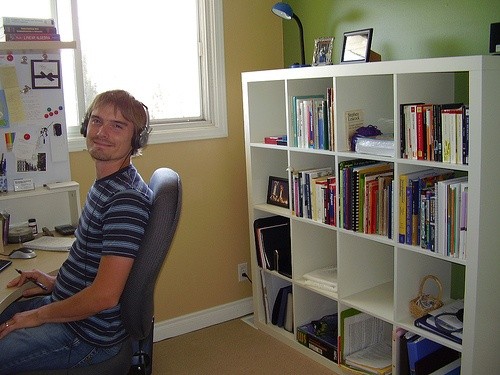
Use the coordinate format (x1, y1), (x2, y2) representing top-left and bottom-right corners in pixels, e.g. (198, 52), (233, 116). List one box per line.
(9, 247), (37, 259)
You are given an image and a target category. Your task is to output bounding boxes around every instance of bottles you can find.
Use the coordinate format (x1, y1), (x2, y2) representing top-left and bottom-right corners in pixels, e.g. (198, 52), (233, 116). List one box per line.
(28, 219), (38, 237)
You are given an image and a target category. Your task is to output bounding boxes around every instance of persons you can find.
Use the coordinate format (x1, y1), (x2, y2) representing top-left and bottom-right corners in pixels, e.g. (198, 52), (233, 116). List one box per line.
(0, 89), (148, 375)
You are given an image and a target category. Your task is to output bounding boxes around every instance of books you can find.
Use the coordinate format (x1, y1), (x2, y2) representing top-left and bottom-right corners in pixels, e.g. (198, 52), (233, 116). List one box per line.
(253, 87), (469, 375)
(0, 17), (60, 42)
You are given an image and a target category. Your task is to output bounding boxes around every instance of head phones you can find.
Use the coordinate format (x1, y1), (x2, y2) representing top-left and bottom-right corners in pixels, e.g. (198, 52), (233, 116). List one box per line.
(81, 102), (150, 149)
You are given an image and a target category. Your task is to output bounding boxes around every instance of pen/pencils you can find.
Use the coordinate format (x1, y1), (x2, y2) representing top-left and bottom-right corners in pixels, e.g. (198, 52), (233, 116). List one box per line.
(0, 153), (7, 172)
(14, 268), (48, 290)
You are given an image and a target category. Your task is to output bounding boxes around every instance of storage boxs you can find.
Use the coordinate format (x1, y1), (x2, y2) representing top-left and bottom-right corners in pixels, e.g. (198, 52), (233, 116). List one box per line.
(296, 313), (338, 363)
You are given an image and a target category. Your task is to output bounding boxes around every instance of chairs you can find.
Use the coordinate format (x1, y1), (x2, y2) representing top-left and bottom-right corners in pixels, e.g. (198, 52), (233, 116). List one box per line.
(10, 167), (184, 375)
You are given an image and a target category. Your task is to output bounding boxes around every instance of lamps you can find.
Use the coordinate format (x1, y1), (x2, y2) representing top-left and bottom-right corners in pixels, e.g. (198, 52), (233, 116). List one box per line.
(272, 2), (311, 69)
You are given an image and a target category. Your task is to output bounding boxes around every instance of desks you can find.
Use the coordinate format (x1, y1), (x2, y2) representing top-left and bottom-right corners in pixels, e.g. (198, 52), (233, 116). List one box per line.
(0, 230), (75, 315)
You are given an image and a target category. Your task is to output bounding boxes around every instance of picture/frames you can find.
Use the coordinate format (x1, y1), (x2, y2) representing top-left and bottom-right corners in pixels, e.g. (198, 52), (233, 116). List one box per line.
(340, 27), (373, 65)
(266, 175), (289, 207)
(312, 36), (335, 67)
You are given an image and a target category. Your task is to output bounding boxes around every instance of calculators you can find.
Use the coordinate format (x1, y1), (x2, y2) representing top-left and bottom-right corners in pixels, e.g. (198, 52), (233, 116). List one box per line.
(55, 224), (75, 236)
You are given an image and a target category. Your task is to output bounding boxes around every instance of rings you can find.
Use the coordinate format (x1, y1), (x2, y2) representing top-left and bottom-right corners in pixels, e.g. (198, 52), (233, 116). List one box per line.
(5, 320), (8, 327)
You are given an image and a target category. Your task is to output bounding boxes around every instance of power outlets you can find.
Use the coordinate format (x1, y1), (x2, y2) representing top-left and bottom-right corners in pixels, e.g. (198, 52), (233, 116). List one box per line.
(237, 262), (249, 282)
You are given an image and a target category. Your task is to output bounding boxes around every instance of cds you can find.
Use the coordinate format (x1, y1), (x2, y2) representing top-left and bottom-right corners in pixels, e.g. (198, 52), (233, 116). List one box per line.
(7, 226), (33, 243)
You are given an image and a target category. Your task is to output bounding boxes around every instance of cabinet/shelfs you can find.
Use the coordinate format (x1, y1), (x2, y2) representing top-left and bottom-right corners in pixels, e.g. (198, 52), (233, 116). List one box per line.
(240, 55), (500, 375)
